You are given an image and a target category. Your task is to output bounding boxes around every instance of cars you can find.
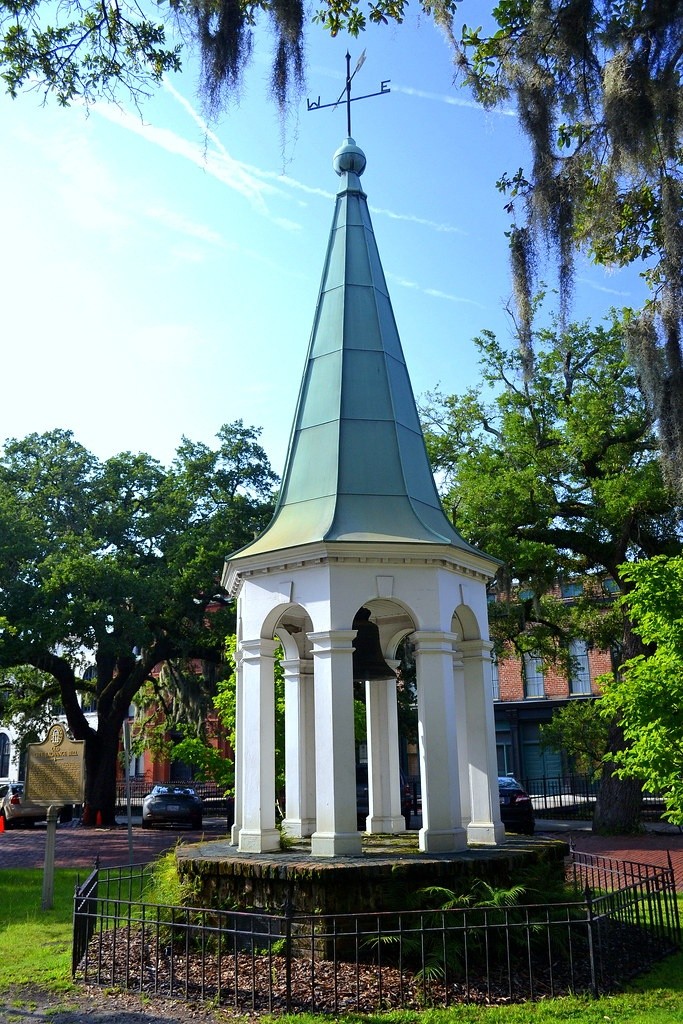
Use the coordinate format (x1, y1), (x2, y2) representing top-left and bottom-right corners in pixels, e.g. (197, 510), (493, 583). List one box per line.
(355, 761), (413, 825)
(0, 779), (46, 828)
(141, 784), (206, 830)
(499, 776), (536, 835)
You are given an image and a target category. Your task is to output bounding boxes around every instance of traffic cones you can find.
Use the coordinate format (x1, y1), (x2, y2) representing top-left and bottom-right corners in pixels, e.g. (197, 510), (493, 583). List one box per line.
(95, 810), (103, 828)
(0, 816), (6, 835)
